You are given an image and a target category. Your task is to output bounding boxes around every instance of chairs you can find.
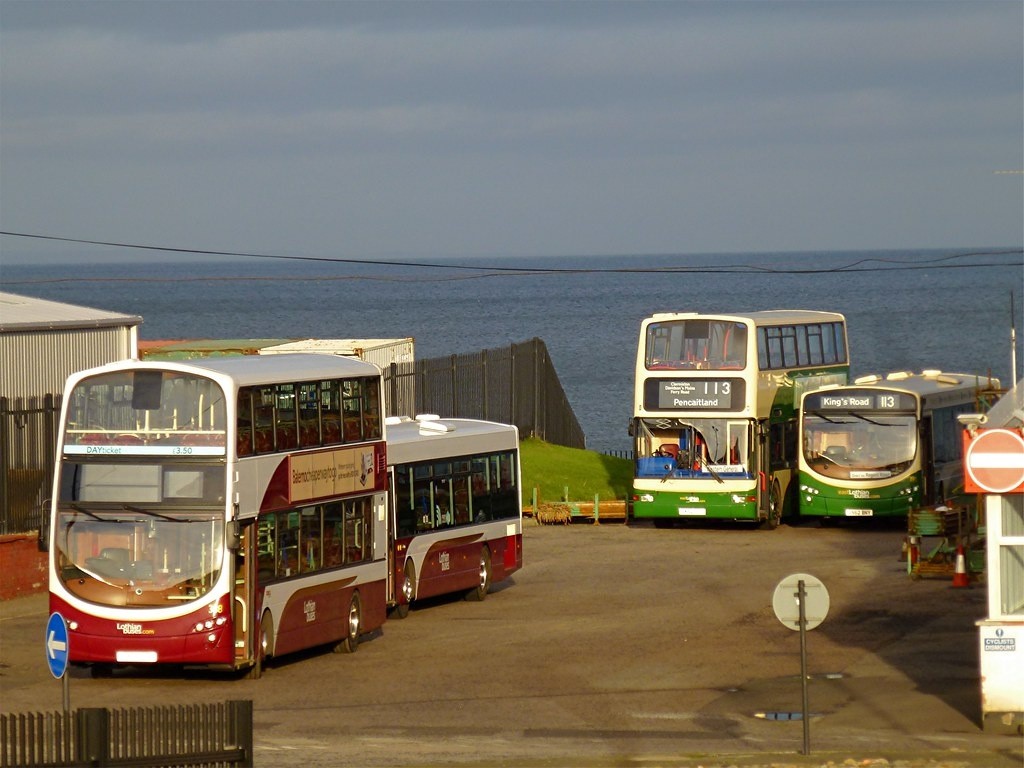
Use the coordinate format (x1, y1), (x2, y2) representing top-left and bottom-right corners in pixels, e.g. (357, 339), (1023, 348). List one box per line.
(183, 435), (211, 441)
(288, 422), (309, 448)
(659, 444), (679, 459)
(75, 425), (111, 446)
(345, 417), (369, 441)
(246, 428), (266, 452)
(307, 423), (321, 446)
(266, 426), (288, 451)
(825, 446), (846, 459)
(415, 472), (497, 533)
(110, 432), (147, 446)
(321, 421), (339, 444)
(137, 519), (369, 575)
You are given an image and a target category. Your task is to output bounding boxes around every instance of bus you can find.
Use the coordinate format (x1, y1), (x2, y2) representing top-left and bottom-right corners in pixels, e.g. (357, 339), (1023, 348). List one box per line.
(386, 418), (522, 620)
(796, 369), (1003, 529)
(37, 353), (388, 678)
(630, 309), (851, 532)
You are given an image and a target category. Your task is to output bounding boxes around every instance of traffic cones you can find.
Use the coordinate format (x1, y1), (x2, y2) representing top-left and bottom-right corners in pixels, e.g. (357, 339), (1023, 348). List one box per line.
(947, 500), (952, 511)
(947, 543), (974, 590)
(897, 537), (908, 562)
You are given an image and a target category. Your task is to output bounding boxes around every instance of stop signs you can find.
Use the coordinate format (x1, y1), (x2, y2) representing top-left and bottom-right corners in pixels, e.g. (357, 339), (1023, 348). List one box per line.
(962, 426), (1024, 495)
